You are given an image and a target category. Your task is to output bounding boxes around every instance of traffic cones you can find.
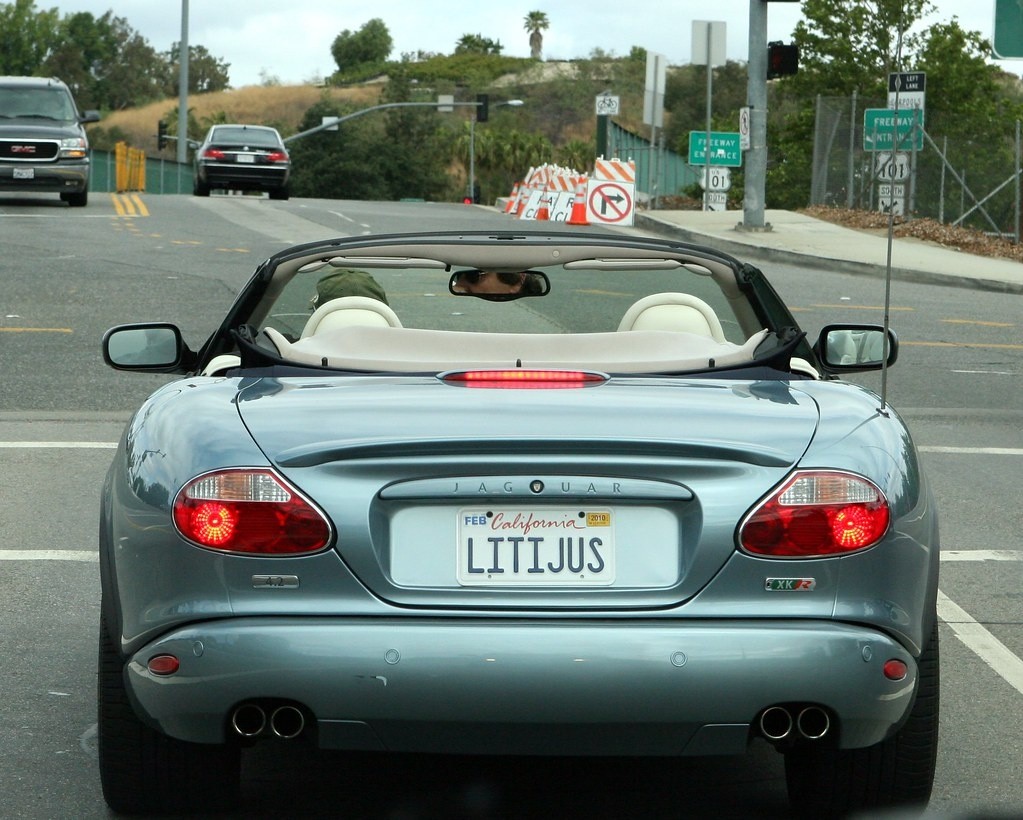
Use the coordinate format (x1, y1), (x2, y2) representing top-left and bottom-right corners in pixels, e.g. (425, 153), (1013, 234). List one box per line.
(565, 176), (592, 226)
(514, 182), (530, 217)
(502, 182), (520, 214)
(534, 185), (549, 222)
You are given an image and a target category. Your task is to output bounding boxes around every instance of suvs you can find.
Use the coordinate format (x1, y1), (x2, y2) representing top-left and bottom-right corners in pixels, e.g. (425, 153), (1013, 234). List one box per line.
(0, 74), (102, 208)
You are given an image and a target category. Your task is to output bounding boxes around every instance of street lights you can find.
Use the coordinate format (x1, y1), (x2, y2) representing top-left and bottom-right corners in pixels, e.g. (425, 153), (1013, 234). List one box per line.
(469, 99), (525, 206)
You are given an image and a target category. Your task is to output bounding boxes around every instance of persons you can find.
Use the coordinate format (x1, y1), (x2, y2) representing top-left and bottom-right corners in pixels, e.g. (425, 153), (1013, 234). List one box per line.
(307, 267), (389, 315)
(452, 273), (544, 293)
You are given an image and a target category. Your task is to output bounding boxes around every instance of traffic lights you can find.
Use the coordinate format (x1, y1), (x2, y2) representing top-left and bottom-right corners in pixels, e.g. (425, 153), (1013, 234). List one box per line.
(158, 121), (168, 151)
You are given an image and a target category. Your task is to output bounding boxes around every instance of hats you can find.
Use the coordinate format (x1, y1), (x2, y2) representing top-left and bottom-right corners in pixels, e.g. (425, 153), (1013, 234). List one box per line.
(308, 270), (388, 306)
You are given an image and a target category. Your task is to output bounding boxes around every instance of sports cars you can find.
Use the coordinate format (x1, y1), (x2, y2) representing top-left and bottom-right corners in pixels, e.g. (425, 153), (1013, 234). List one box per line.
(94, 229), (942, 820)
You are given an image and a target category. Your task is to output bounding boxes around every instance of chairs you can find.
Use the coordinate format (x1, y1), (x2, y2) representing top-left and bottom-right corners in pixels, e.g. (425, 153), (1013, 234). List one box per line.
(300, 296), (404, 338)
(618, 292), (725, 342)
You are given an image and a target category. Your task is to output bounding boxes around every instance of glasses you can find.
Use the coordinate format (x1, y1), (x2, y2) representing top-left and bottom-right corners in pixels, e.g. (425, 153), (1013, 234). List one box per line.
(453, 271), (521, 285)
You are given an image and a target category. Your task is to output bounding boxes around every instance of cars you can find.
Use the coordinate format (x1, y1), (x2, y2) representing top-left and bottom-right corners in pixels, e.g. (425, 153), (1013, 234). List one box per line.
(190, 123), (291, 200)
